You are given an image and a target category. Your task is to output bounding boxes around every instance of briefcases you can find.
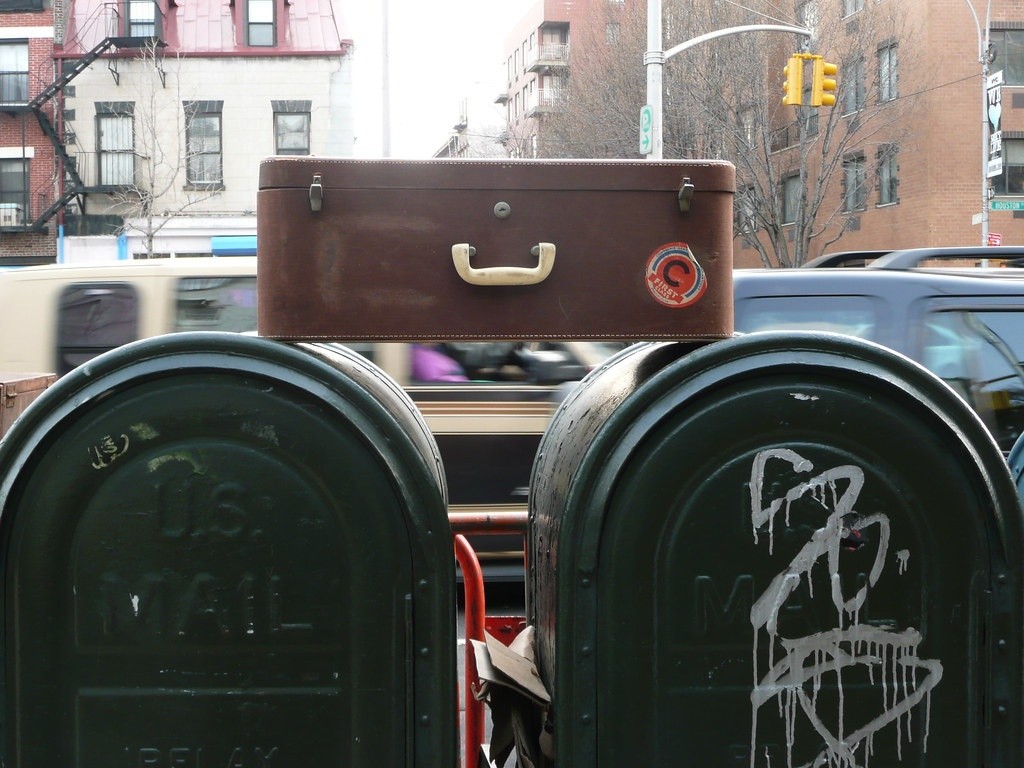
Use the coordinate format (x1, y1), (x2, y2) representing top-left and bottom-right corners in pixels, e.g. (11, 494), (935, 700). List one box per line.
(257, 155), (736, 342)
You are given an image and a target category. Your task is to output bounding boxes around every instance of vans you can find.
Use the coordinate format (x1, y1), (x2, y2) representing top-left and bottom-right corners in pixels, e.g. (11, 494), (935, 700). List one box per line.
(1, 249), (616, 585)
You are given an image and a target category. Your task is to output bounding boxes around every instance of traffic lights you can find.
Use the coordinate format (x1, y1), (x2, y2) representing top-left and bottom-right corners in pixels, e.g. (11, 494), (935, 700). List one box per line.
(781, 55), (803, 108)
(809, 58), (839, 108)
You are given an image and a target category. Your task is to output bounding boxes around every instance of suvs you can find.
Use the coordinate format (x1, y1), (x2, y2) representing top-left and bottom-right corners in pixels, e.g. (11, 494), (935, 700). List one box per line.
(733, 246), (1024, 463)
